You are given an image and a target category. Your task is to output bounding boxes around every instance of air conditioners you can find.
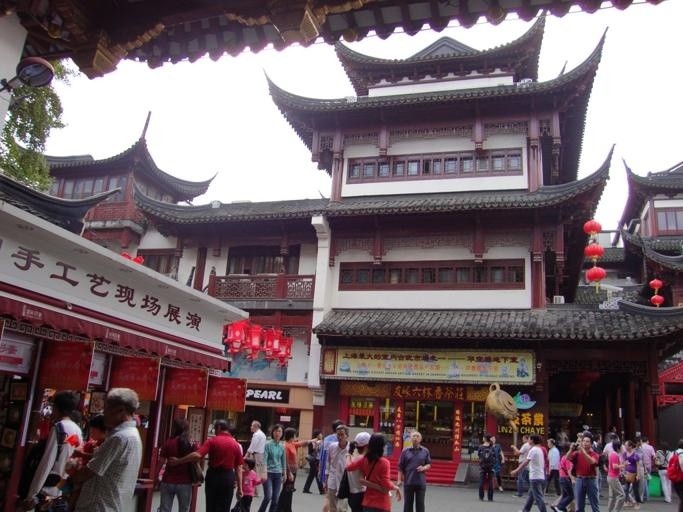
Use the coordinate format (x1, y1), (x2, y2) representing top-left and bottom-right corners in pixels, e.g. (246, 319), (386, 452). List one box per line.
(553, 294), (565, 306)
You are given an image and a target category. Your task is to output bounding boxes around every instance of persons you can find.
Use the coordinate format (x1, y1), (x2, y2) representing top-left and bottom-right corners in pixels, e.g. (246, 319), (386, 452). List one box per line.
(71, 386), (143, 511)
(230, 458), (262, 512)
(168, 418), (243, 512)
(40, 395), (52, 415)
(478, 433), (504, 502)
(317, 421), (432, 512)
(22, 391), (83, 512)
(65, 416), (104, 511)
(245, 420), (324, 511)
(510, 431), (683, 511)
(152, 416), (204, 511)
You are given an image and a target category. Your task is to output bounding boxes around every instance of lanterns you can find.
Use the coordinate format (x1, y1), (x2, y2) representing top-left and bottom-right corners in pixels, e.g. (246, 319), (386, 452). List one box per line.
(583, 241), (602, 266)
(651, 295), (664, 308)
(649, 279), (663, 294)
(587, 267), (605, 294)
(120, 252), (131, 260)
(133, 256), (144, 264)
(583, 220), (601, 236)
(224, 320), (292, 371)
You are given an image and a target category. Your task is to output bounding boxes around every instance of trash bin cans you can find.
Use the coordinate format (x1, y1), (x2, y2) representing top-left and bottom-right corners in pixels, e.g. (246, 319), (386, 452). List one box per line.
(646, 472), (661, 497)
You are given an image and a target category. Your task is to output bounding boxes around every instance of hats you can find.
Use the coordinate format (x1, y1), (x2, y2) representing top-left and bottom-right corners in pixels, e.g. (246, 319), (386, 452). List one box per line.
(353, 432), (370, 447)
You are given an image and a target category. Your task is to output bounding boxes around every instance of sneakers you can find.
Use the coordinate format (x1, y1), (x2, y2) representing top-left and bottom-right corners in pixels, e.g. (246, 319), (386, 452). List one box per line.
(551, 503), (567, 512)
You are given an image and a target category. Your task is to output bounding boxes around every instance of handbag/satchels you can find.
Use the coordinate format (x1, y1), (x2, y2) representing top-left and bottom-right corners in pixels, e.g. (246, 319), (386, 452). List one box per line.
(618, 471), (637, 485)
(337, 470), (350, 499)
(191, 462), (204, 486)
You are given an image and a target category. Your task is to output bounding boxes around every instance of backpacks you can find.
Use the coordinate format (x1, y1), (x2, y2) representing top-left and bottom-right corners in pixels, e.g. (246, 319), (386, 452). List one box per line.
(666, 449), (683, 484)
(478, 445), (495, 470)
(17, 422), (66, 497)
